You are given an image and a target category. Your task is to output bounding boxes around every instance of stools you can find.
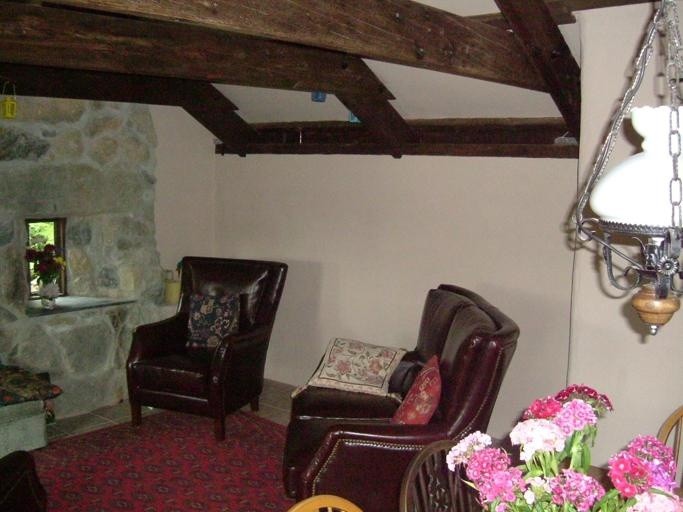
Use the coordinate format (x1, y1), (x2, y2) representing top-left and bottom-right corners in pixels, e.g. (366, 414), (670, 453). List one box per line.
(0, 364), (64, 459)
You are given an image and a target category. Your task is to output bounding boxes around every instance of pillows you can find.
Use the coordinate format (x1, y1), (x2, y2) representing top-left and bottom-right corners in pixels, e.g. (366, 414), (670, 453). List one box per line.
(307, 337), (407, 396)
(185, 295), (241, 351)
(390, 355), (442, 425)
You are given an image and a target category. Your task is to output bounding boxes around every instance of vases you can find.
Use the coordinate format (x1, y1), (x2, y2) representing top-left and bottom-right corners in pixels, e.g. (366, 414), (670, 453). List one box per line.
(41, 299), (55, 309)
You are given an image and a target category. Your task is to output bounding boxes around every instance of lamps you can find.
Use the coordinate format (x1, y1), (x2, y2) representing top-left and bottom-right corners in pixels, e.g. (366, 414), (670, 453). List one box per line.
(571, 0), (683, 335)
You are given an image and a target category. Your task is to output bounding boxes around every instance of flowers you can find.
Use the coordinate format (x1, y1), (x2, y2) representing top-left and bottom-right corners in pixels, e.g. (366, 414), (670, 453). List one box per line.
(446, 383), (683, 512)
(24, 235), (68, 299)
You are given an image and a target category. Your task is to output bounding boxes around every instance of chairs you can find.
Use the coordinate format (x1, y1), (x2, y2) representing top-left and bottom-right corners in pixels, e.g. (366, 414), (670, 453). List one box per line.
(657, 406), (683, 487)
(126, 256), (289, 441)
(399, 440), (479, 512)
(282, 284), (520, 512)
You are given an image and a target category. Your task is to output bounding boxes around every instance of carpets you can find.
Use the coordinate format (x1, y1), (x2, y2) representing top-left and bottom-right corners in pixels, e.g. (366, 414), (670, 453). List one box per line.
(27, 410), (297, 512)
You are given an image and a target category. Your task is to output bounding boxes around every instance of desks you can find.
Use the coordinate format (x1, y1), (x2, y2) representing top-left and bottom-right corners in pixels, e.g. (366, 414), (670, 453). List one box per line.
(527, 456), (683, 500)
